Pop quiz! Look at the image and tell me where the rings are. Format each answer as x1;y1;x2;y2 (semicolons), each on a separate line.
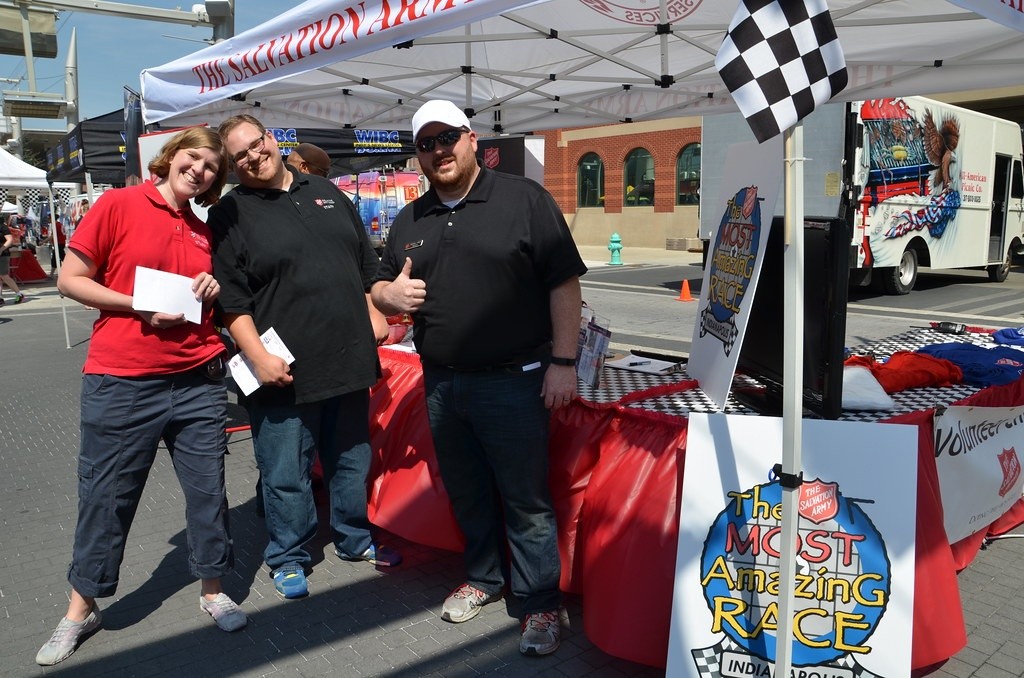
209;286;213;290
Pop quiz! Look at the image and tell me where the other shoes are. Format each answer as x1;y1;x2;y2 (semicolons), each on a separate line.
46;276;54;281
256;501;265;516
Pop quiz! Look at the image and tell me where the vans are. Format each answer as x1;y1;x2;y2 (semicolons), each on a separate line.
849;95;1024;296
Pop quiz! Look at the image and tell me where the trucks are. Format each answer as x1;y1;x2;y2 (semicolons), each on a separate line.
33;195;103;244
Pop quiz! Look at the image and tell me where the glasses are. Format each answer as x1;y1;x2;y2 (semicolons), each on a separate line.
304;160;329;179
230;132;265;167
415;129;469;153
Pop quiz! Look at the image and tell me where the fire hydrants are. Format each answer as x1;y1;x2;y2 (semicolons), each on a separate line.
608;233;623;264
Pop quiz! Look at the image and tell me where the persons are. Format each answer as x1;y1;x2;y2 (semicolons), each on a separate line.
36;126;246;666
0;222;24;306
39;214;66;280
371;90;586;656
287;143;330;178
206;113;402;598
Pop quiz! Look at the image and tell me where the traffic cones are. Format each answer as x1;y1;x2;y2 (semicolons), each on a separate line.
675;280;696;301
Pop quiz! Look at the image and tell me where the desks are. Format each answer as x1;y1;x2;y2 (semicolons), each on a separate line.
312;326;1024;670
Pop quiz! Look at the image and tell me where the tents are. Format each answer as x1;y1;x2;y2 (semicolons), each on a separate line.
139;1;1024;678
47;108;125;270
0;146;103;271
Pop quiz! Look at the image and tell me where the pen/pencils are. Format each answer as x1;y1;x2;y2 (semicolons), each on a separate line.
626;361;651;366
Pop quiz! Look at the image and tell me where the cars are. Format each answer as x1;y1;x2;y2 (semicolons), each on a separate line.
8;216;35;245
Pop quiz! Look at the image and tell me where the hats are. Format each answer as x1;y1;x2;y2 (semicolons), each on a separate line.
295;143;331;178
412;100;473;147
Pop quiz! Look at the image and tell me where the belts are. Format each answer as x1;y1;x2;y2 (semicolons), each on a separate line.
425;362;522;376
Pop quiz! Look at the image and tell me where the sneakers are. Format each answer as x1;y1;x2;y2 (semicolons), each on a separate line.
0;299;5;307
519;608;560;656
15;294;24;303
335;542;401;567
273;567;307;598
36;599;102;666
440;583;503;623
200;590;247;633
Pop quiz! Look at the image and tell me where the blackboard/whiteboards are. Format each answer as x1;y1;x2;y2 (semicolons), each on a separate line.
699;102;846;239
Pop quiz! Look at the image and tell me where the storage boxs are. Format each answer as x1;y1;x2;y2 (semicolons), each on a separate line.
1;246;21;258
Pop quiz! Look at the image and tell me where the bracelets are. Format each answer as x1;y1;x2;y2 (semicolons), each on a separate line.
551;356;576;365
